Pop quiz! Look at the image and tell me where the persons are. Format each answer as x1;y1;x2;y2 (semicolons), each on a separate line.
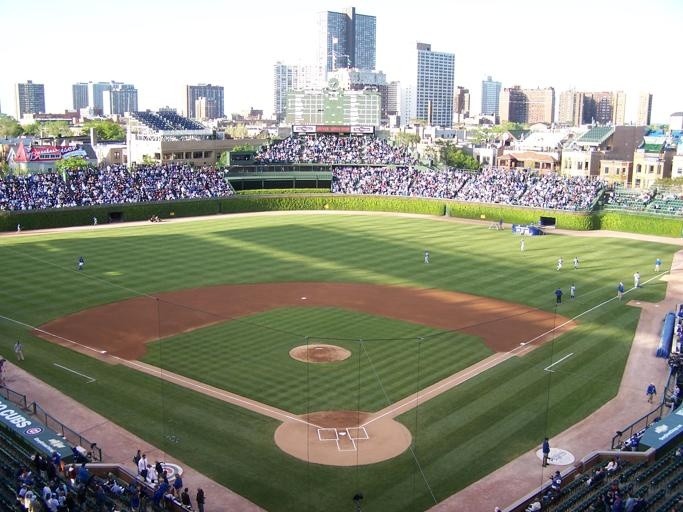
633;272;640;288
0;338;207;511
573;256;579;268
557;257;564;270
0;160;233;212
17;224;21;231
494;307;683;512
654;258;661;271
425;250;428;263
570;284;576;298
93;217;98;226
617;282;624;301
255;134;419;166
520;239;525;251
333;166;683;212
554;287;563;305
78;256;84;270
